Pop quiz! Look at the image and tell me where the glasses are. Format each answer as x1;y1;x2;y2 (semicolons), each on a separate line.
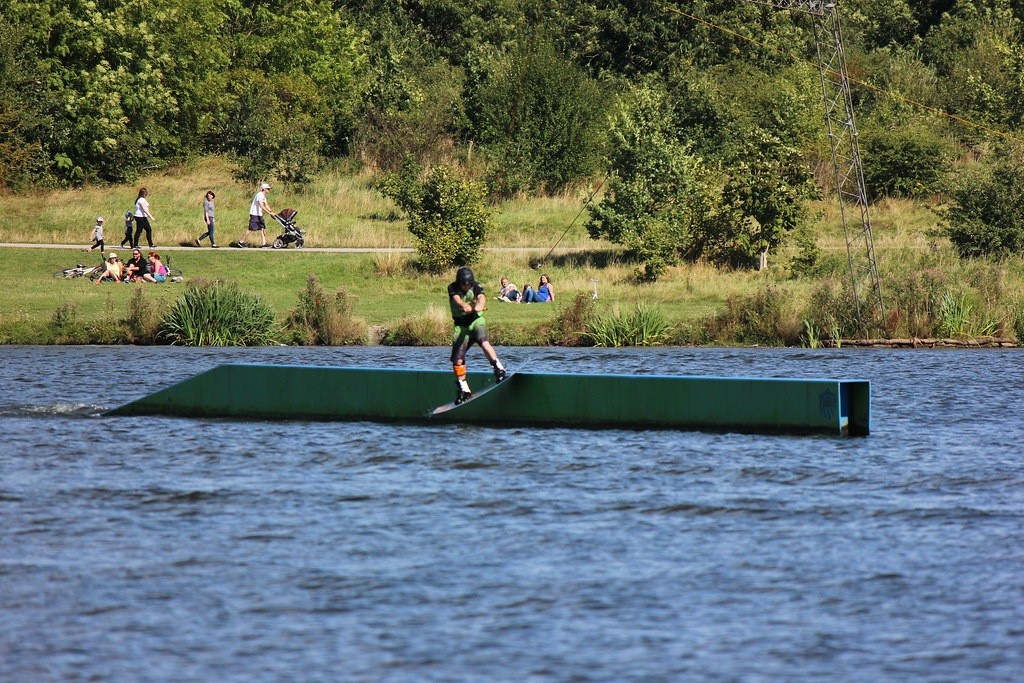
148;256;150;259
133;253;140;255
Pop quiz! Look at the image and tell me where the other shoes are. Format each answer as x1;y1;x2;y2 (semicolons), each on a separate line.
490;358;506;384
212;245;219;248
455;379;471;405
196;239;200;247
86;243;167;284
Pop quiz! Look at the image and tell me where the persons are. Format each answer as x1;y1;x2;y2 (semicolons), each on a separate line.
237;184;276;248
446;267;507;406
86;217;105;253
195;190;219;248
497;277;523;304
521;273;555;304
123;249;147;283
290;221;306;234
522;284;532;295
120;211;137;249
143;252;167;282
133;187;158;249
95;252;124;284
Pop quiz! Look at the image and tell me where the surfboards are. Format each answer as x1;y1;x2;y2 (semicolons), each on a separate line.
432;356;532;415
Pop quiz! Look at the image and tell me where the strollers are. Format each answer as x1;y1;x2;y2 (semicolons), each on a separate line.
271;208;307;248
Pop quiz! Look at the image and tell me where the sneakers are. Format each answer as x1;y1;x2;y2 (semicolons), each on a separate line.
498;296;531;304
238;241;247;248
262;244;272;248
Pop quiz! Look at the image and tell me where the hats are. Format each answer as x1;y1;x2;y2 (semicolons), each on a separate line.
125;212;131;217
97;217;103;221
261;184;271;189
109;253;117;258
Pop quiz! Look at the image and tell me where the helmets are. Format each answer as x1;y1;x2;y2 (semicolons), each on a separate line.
456;267;474;285
